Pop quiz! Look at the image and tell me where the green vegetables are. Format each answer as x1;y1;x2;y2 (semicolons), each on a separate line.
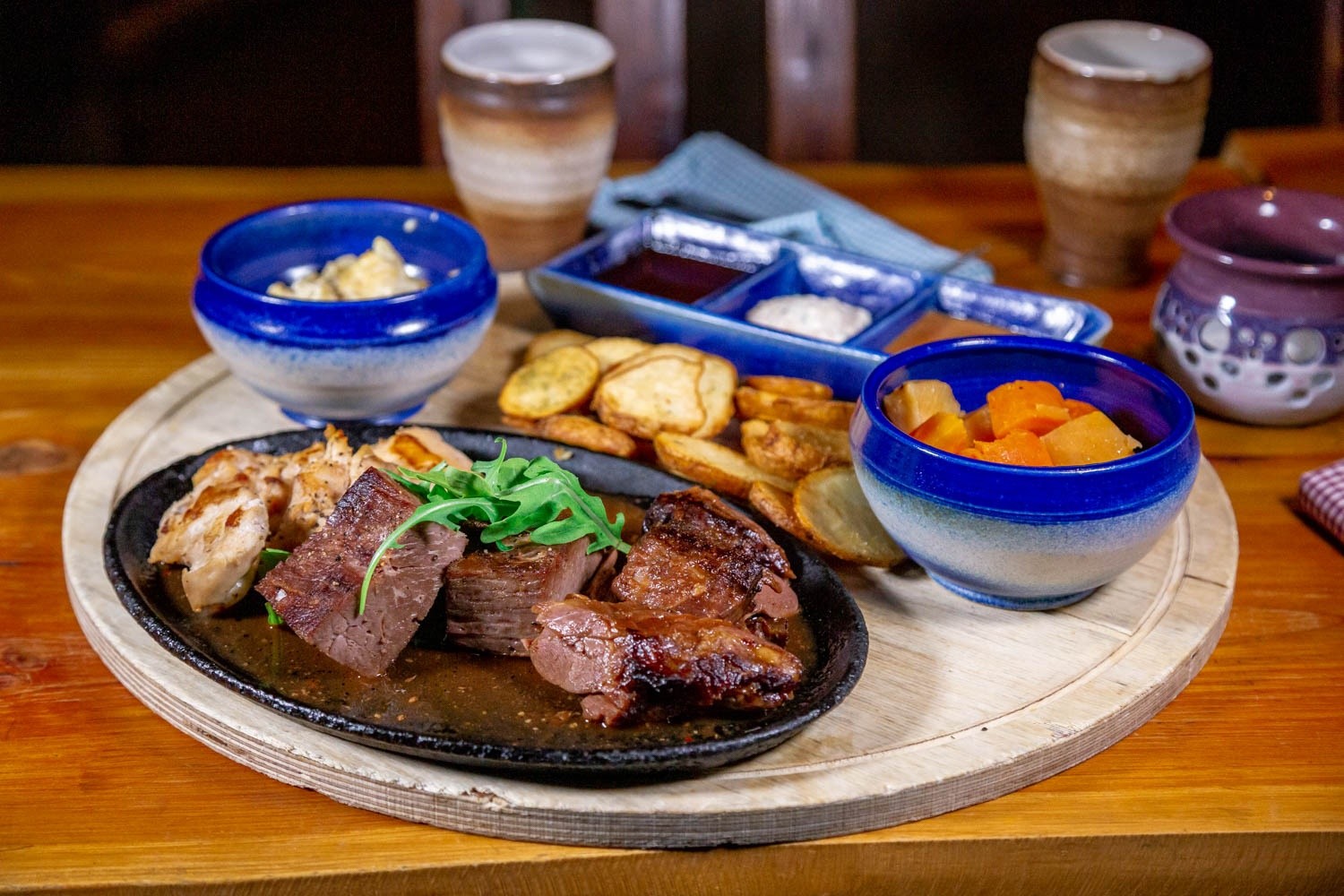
251;432;631;623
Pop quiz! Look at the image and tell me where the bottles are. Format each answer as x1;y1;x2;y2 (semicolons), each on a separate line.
1152;186;1344;430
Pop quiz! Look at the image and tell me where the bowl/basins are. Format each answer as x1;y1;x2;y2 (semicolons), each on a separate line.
525;201;1114;404
188;197;497;427
848;333;1200;609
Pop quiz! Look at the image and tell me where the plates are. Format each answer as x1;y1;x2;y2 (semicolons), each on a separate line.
103;427;870;785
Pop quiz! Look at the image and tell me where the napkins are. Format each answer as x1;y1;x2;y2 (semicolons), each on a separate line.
587;133;993;285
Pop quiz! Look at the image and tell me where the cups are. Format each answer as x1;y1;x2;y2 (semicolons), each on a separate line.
1024;20;1215;288
437;17;621;273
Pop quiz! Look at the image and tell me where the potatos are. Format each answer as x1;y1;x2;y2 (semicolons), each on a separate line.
494;331;910;568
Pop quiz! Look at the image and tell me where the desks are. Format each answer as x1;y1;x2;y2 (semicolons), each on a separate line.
0;161;1344;896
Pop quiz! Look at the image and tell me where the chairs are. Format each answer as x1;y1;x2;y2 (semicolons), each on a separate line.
416;0;860;163
